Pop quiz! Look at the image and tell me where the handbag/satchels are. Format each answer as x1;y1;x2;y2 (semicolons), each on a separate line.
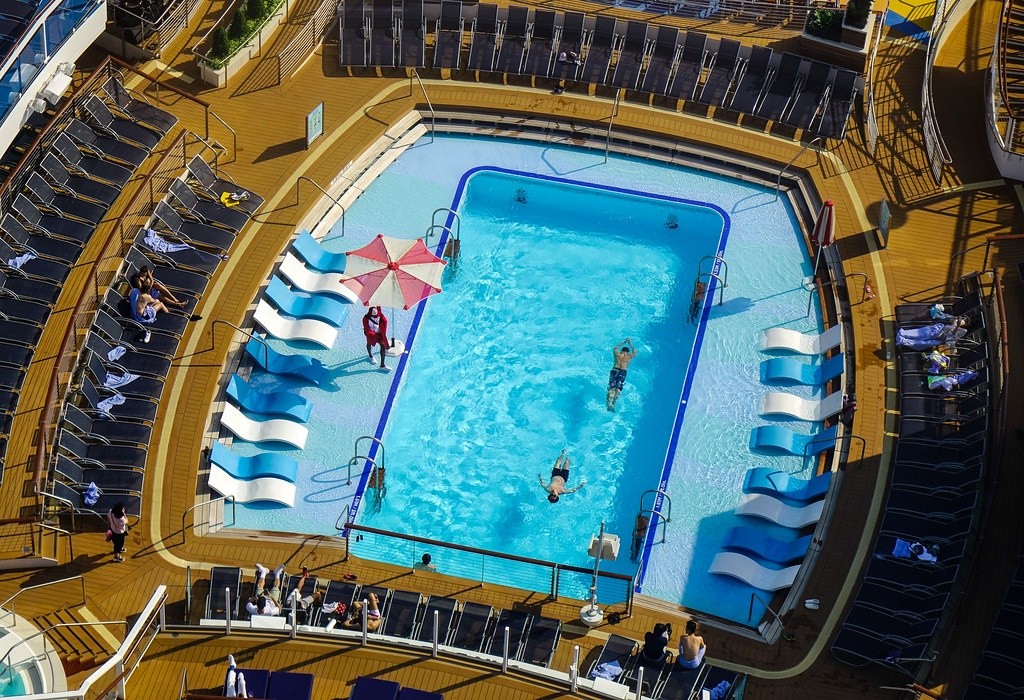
124;526;129;536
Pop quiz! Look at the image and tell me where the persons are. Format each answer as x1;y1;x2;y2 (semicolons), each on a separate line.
226;654;248;698
642;623;672;664
606;338;638;413
363;307;392;371
895;315;970;350
129;266;188;323
230;190;248;201
631;515;649;560
343;592;380;631
538;449;585;503
677;621;706;669
108;502;129;562
553;85;565;93
559;50;581;65
416;553;436;572
247;563;322;616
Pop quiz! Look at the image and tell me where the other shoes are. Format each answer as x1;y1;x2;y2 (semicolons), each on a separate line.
805;599;819;604
369;356;378;364
255;564;270;574
343;573;359;581
303;567;308;578
805;604;819;609
274;564;284;575
380;365;392;371
113;555;124;562
783;632;795;641
665;623;672;635
120;547;126;552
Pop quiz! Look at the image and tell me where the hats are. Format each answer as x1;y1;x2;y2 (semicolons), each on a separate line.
369;306;377;315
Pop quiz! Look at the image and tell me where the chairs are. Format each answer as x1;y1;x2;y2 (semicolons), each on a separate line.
1;1;1024;700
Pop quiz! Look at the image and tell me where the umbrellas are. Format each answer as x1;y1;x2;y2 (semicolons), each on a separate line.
812;200;835;282
339;236;448;348
